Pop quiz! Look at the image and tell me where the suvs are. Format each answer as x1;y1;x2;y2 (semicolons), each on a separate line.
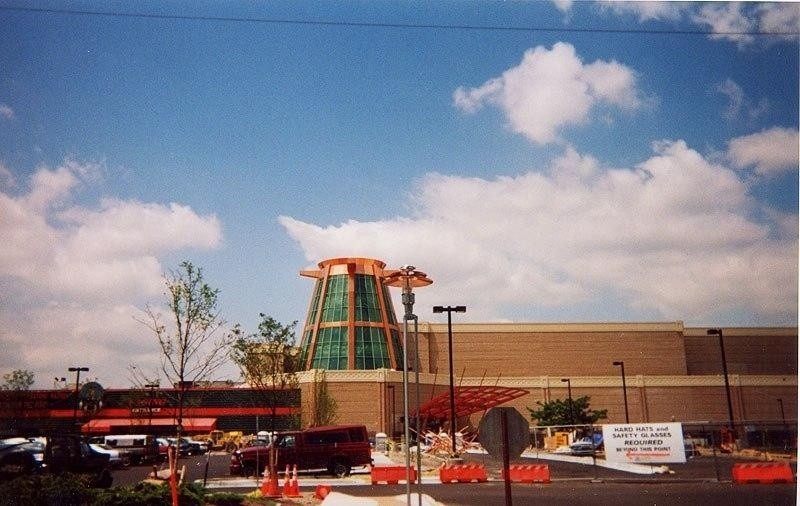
227;422;372;478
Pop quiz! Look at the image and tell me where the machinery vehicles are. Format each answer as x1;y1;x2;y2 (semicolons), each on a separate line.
194;429;254;452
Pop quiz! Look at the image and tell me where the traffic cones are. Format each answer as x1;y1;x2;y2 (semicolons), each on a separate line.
291;465;303;498
260;467;272;498
282;462;292;498
269;465;281;498
315;485;332;499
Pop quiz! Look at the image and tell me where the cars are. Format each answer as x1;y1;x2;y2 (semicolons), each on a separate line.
571;436;597;449
0;431;212;483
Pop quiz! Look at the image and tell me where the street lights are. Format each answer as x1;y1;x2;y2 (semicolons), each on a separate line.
55;377;67;382
777;398;789;447
558;379;575;434
143;384;159;433
430;304;467;458
706;328;739;446
67;366;90;444
613;360;632;423
382;262;435;505
